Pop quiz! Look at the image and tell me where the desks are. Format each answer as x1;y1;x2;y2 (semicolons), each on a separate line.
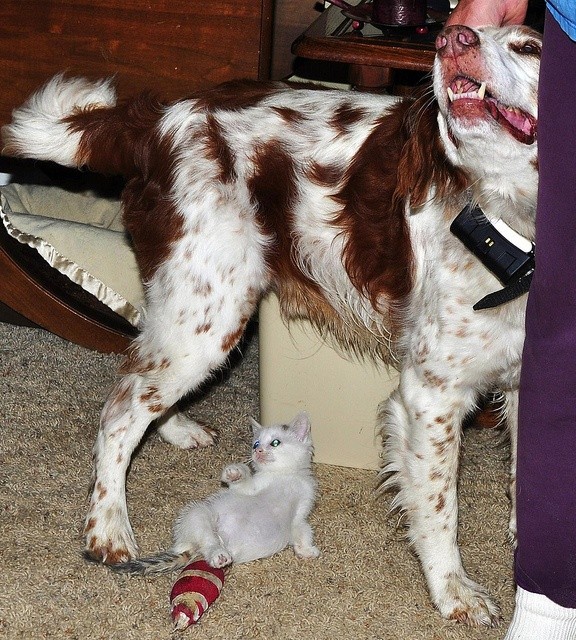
290;0;466;91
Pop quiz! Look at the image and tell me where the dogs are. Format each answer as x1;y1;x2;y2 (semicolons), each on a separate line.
2;21;545;630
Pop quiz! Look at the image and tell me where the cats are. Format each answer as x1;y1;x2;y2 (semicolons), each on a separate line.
107;411;323;579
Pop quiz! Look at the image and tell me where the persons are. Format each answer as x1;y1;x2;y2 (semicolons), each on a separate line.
444;1;576;640
